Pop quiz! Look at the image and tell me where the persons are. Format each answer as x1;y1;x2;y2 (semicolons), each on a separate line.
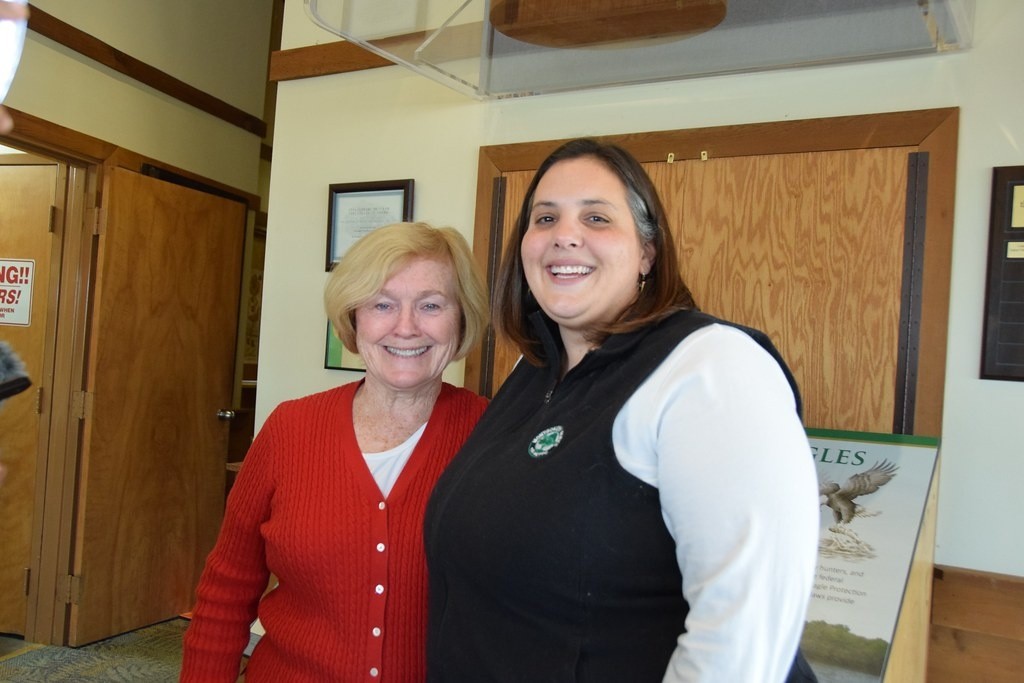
176;222;490;683
420;139;817;681
